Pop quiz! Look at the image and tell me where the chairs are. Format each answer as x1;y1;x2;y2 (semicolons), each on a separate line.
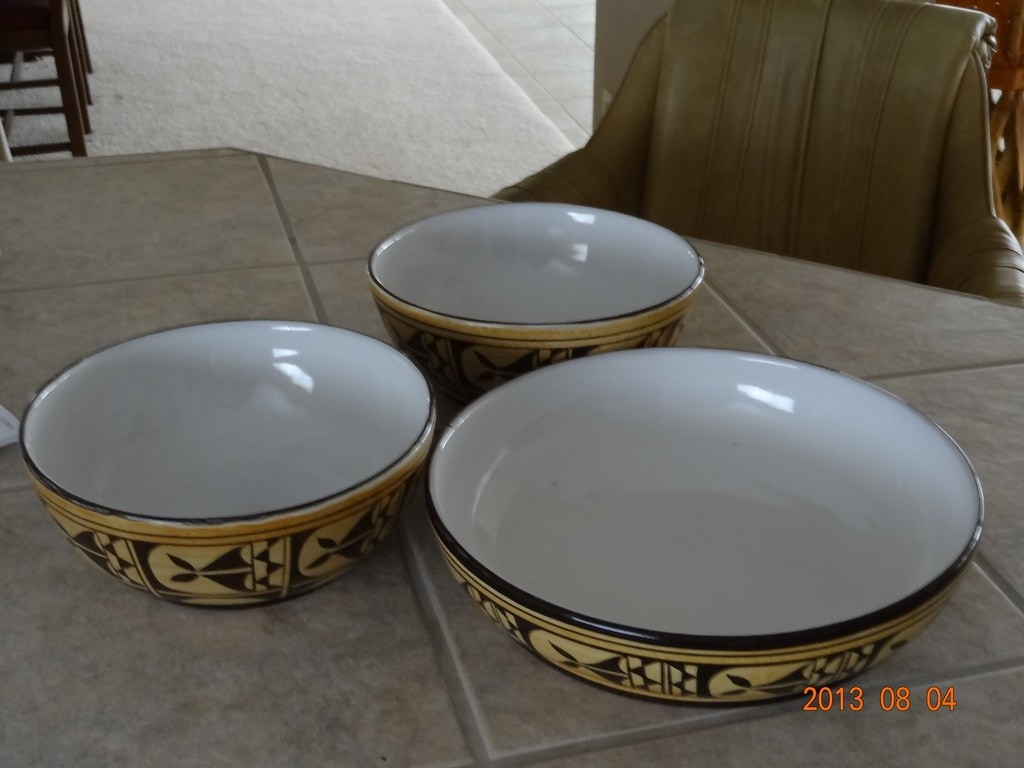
489;0;1024;304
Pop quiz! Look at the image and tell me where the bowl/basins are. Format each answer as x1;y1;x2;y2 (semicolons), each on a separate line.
19;319;435;612
367;201;706;400
423;344;989;711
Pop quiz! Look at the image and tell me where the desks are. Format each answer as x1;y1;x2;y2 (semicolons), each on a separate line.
0;148;1024;768
